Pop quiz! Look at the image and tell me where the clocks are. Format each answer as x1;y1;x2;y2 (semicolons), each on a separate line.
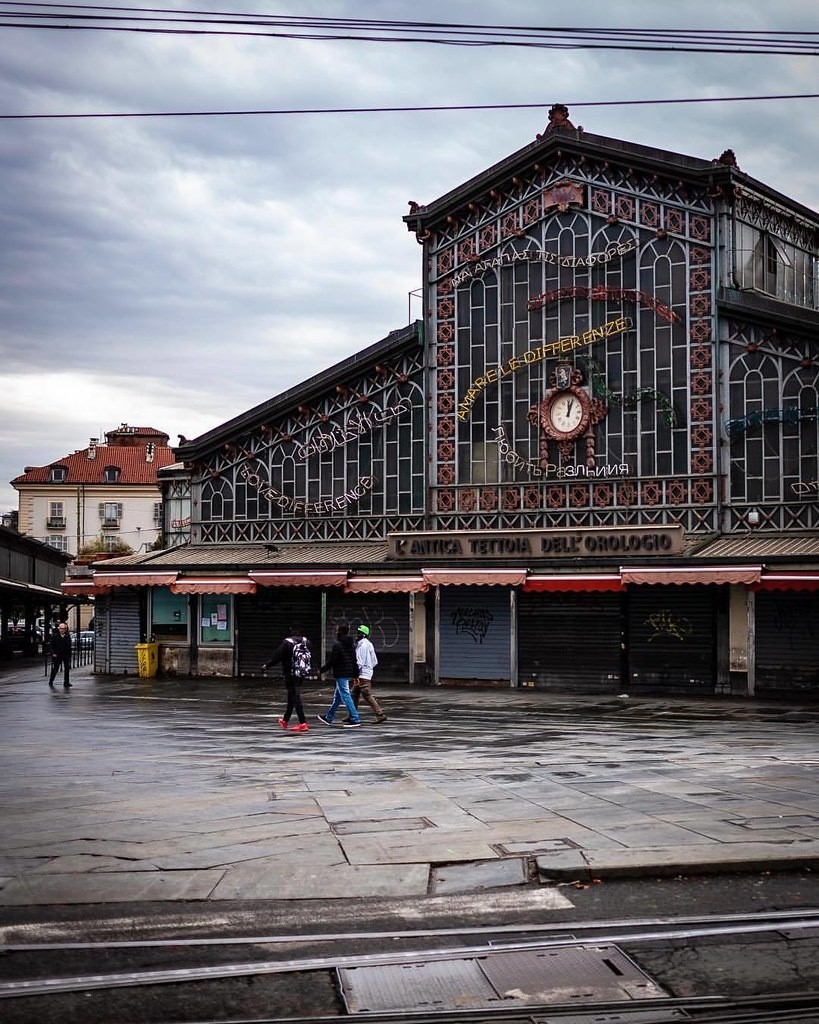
550;392;585;433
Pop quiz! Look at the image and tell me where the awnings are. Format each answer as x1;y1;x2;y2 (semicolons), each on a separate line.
93;570;178;586
522;572;626;592
60;580;112;596
746;571;819;593
248;569;350;586
622;565;763;584
344;575;429;594
170;577;257;594
421;568;528;586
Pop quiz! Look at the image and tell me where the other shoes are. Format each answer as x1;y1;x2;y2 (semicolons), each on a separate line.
371;714;387;724
48;682;54;688
342;717;352;722
64;683;73;688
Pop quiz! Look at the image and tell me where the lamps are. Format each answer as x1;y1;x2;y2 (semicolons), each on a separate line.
263;543;280;558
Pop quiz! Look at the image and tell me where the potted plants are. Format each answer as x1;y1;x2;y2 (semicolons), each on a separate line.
77;531;135;561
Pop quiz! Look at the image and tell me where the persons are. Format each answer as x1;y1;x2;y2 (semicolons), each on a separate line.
317;624;361;728
12;625;21;632
48;623;73;688
261;623;314;731
342;624;387;724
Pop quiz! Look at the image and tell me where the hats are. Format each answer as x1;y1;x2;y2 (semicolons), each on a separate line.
357;625;370;636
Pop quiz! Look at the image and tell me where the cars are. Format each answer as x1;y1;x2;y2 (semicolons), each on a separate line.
6;616;95;655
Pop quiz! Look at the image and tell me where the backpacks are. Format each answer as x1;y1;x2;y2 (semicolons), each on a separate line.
284;636;312;677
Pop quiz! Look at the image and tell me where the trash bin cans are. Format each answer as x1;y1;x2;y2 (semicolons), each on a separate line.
134;643;160;678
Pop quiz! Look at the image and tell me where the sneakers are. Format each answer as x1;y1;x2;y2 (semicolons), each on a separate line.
317;715;341;721
343;721;361;727
278;719;289;728
290;722;309;731
